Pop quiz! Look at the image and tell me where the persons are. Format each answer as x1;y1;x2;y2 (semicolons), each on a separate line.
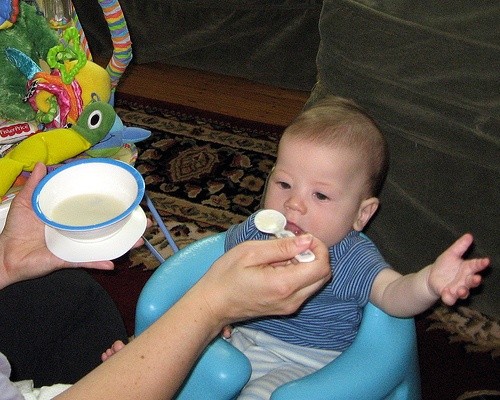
0;162;332;400
102;95;490;400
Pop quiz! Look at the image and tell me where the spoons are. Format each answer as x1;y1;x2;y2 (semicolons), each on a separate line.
254;208;316;263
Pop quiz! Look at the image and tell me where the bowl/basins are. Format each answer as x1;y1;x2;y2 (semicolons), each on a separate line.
31;158;145;242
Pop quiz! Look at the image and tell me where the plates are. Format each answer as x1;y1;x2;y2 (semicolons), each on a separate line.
44;205;147;262
